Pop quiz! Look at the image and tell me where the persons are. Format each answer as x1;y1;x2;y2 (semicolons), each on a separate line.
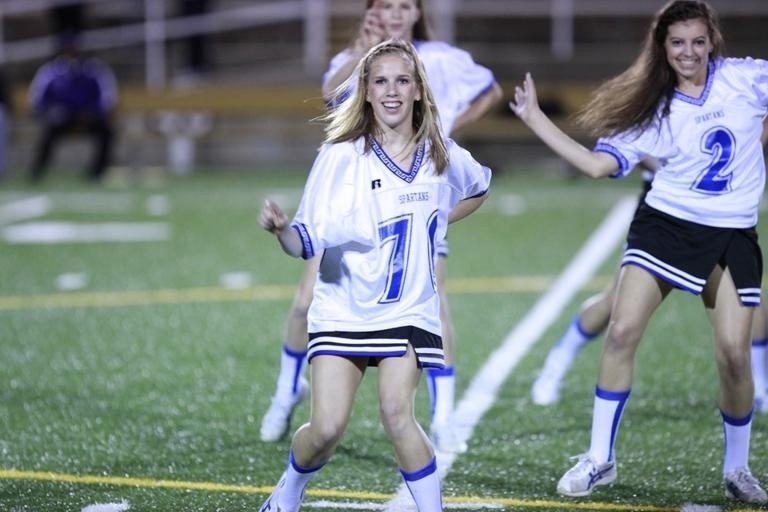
258;34;490;509
256;2;503;457
161;54;223;173
525;252;766;416
509;0;766;508
25;34;116;183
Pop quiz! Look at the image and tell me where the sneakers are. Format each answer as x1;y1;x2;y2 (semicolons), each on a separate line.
258;472;308;512
557;448;618;498
259;378;309;441
427;423;466;454
720;468;767;506
533;347;573;406
